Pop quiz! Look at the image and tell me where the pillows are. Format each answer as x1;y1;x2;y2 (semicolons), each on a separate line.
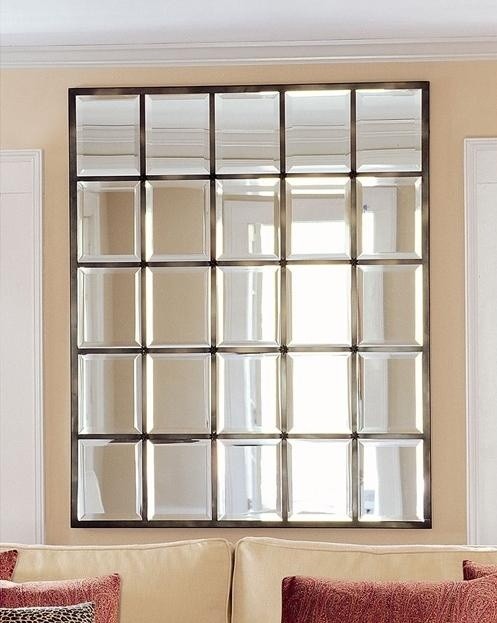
0;547;123;623
280;558;497;623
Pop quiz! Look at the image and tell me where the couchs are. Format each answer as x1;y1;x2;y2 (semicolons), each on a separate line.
0;534;497;623
